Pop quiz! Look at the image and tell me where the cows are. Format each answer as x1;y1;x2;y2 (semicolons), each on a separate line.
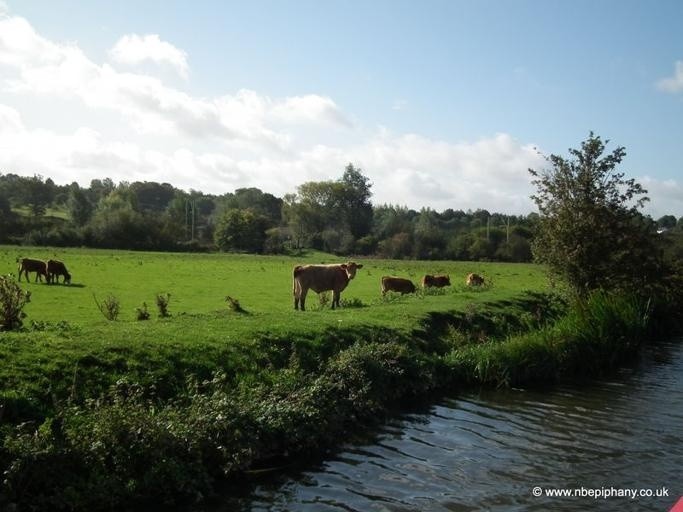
466;273;484;286
18;258;52;283
293;262;364;311
421;274;452;289
47;259;71;285
381;276;417;297
